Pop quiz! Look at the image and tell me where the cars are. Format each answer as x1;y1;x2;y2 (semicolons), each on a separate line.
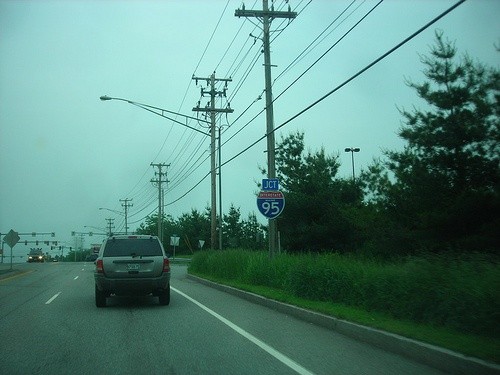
52;258;58;262
27;249;45;263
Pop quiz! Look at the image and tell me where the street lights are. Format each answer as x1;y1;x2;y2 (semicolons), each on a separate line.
345;146;360;180
85;225;112;237
100;94;222;250
99;207;128;235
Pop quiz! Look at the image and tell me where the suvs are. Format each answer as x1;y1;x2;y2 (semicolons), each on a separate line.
93;233;172;308
